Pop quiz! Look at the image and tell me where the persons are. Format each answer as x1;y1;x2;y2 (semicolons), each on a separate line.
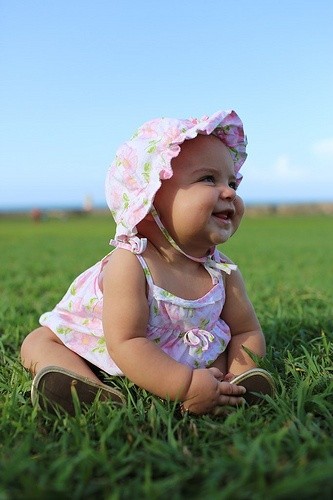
20;108;277;418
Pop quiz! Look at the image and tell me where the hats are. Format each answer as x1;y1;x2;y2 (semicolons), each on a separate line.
104;108;249;245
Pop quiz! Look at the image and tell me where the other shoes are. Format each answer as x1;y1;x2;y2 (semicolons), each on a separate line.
226;369;277;411
30;367;128;417
